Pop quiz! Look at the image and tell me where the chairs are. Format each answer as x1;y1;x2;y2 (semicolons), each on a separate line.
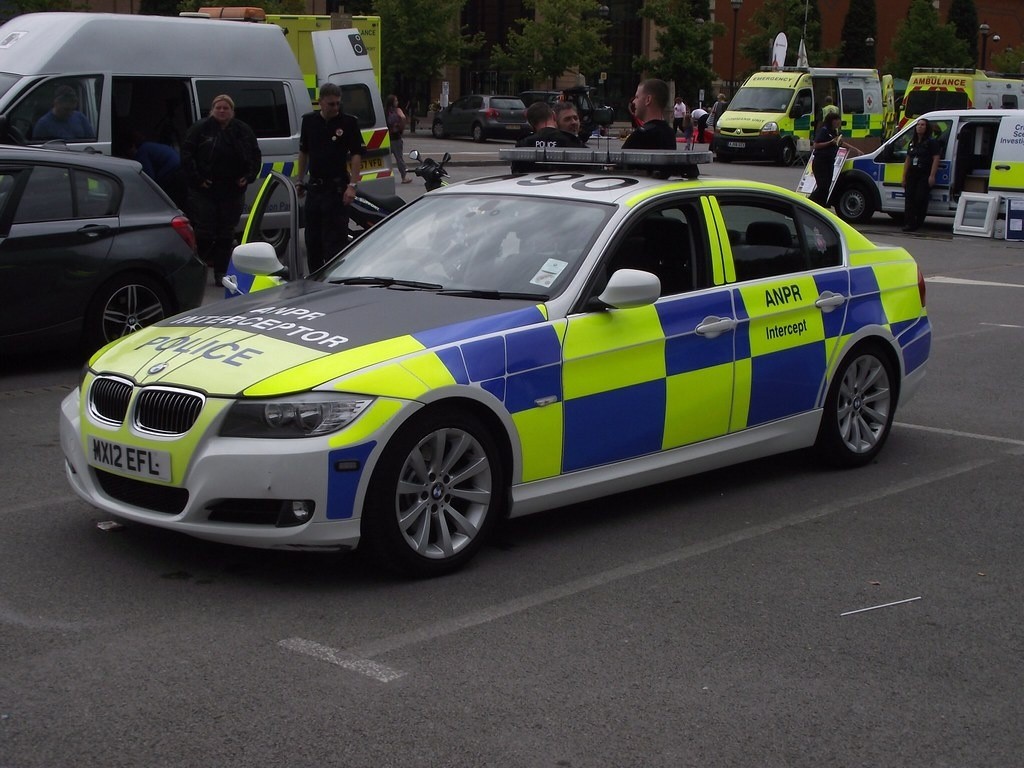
32;170;92;219
791;136;810;168
746;221;793;249
639;216;695;297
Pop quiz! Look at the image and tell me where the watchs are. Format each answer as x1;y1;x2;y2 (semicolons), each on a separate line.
347;183;359;192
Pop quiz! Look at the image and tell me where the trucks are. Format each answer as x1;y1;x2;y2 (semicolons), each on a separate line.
180;7;382;110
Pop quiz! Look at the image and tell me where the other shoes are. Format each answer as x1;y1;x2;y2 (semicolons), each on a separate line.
401;178;412;183
214;273;226;287
416;119;420;124
902;224;917;232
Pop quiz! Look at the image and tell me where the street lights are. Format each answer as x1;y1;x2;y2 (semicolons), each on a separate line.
730;0;745;102
552;2;610;91
979;22;1014;74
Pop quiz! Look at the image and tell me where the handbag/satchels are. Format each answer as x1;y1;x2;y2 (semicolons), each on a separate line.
705;115;714;126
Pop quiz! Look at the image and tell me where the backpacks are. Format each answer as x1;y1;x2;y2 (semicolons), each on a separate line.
387;107;401;134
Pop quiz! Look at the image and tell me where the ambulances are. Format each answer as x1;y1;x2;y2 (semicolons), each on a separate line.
898;65;1024;135
709;66;896;166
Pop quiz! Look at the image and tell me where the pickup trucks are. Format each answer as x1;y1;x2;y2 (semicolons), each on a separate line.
520;89;614;144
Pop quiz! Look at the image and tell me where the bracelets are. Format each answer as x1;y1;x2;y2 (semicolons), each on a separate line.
295;177;303;187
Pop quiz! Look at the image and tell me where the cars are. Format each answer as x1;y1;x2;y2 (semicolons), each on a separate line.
0;139;204;377
432;94;533;142
55;157;933;576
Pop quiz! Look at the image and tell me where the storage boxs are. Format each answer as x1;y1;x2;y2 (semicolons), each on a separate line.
797;139;810;151
964;174;989;193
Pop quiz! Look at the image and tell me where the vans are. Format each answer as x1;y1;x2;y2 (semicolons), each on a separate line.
832;109;1024;225
0;12;318;259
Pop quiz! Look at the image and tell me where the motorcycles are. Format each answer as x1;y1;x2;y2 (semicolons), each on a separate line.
345;148;453;244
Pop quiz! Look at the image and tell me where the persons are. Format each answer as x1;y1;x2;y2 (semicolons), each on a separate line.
32;85;95;196
182;94;262;287
511;101;582;174
120;130;182;200
808;96;841;208
294;83;366;274
385;94;412;184
621;79;677;150
405;96;420;125
902;118;941;232
673;93;728;151
628;95;638;132
